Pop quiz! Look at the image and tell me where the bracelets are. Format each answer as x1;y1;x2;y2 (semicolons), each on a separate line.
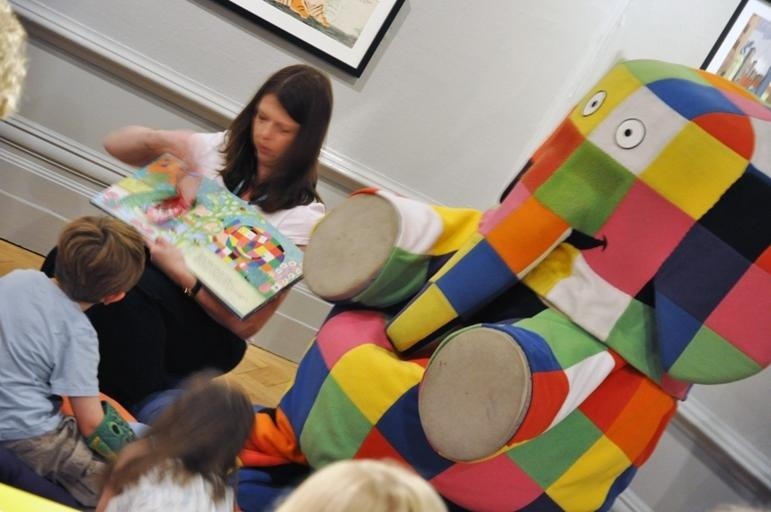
144;130;158;152
182;278;201;298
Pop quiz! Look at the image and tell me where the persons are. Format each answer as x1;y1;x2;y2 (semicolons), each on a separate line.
36;64;337;424
272;455;451;511
103;376;254;512
0;210;145;510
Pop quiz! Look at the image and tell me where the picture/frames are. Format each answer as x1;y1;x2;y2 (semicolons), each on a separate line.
698;0;771;110
210;0;405;79
